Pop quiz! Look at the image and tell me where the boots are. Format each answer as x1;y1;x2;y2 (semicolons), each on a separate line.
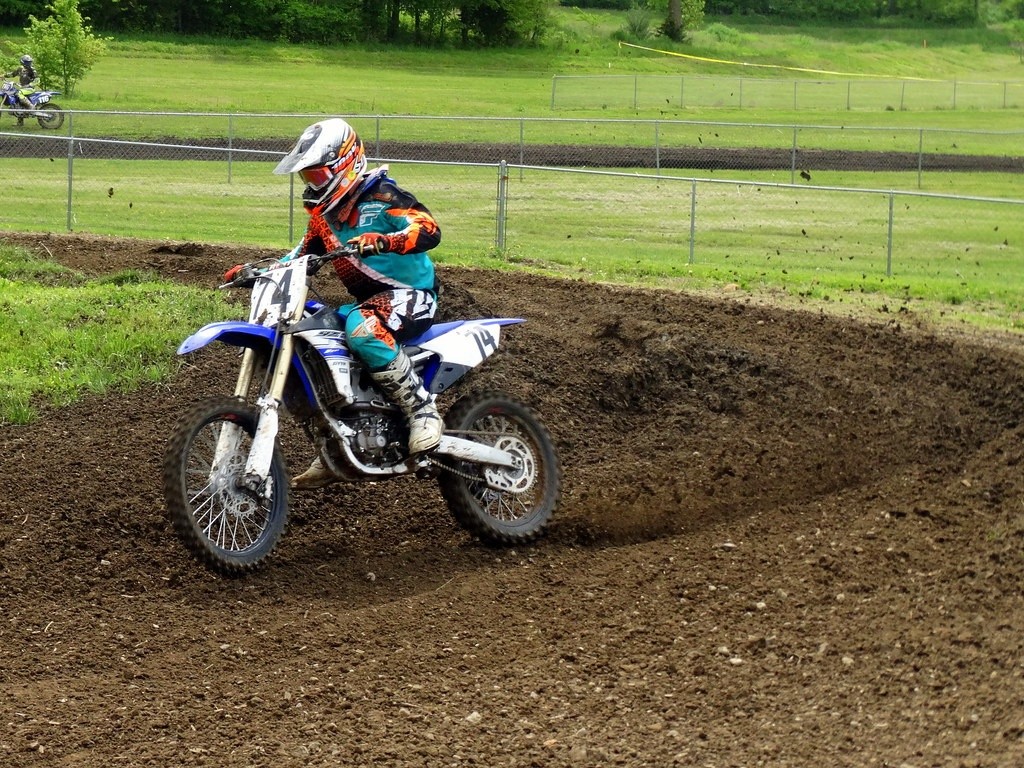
293;452;333;485
370;347;445;454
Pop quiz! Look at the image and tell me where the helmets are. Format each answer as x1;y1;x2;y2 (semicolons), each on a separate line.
272;118;367;218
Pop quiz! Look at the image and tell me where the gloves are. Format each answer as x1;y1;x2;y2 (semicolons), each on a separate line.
346;232;389;256
225;264;243;281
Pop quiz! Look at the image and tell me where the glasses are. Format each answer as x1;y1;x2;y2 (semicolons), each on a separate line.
297;135;360;191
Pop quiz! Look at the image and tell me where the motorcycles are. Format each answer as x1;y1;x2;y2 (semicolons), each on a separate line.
0;73;65;129
161;240;561;577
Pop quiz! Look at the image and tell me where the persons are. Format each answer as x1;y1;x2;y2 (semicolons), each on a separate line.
0;55;42;126
222;118;447;490
